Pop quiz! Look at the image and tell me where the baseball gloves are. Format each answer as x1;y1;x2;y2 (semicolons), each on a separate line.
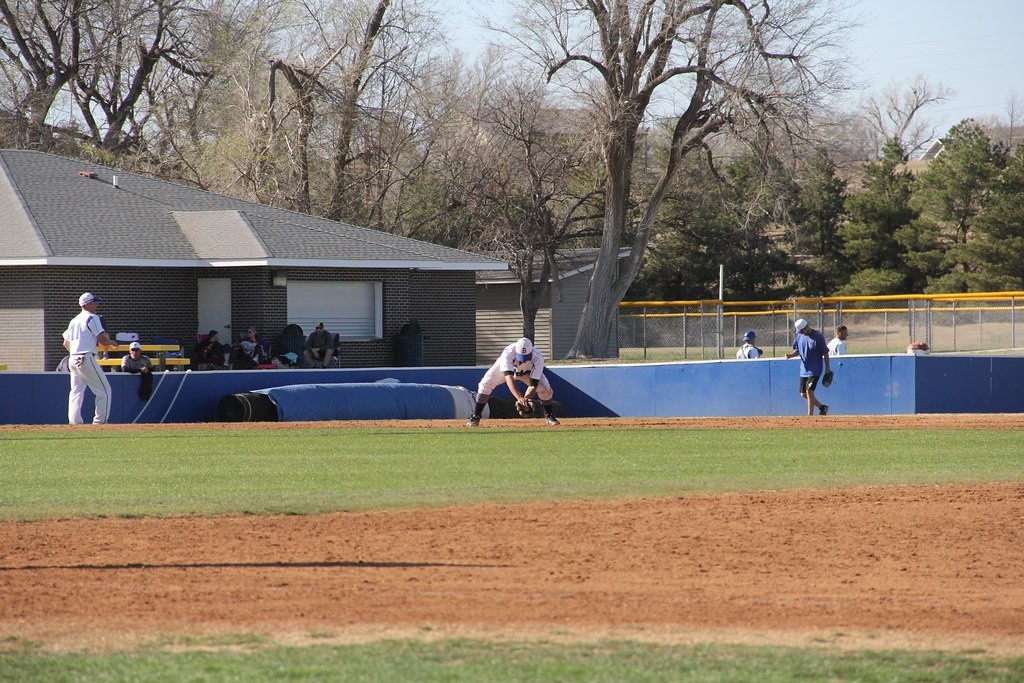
514;398;536;419
822;371;833;387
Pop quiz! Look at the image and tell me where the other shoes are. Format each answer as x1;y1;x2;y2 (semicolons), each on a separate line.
818;405;829;415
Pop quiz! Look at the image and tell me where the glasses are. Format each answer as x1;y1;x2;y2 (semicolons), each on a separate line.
246;330;253;334
316;326;323;329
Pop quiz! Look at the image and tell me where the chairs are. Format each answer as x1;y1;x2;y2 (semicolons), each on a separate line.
115;331;140;357
238;334;270;368
320;332;341;366
152;337;185;373
196;333;222;370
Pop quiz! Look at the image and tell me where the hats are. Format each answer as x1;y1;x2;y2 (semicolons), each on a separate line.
129;342;141;350
515;338;532;361
78;292;101;307
742;332;755;341
795;318;807;333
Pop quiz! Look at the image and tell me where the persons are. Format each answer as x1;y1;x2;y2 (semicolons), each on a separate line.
785;319;833;415
62;292;118;424
737;331;758;359
468;337;560;427
827;326;848;356
121;342;153;373
304;323;335;368
195;328;261;370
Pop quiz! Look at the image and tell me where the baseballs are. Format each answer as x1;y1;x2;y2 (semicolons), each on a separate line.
520;405;529;412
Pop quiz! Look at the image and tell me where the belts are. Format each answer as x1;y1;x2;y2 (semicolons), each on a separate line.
79;353;95;356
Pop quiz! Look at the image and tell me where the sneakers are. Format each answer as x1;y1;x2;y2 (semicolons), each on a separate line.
467;415;481;427
545;417;559;426
